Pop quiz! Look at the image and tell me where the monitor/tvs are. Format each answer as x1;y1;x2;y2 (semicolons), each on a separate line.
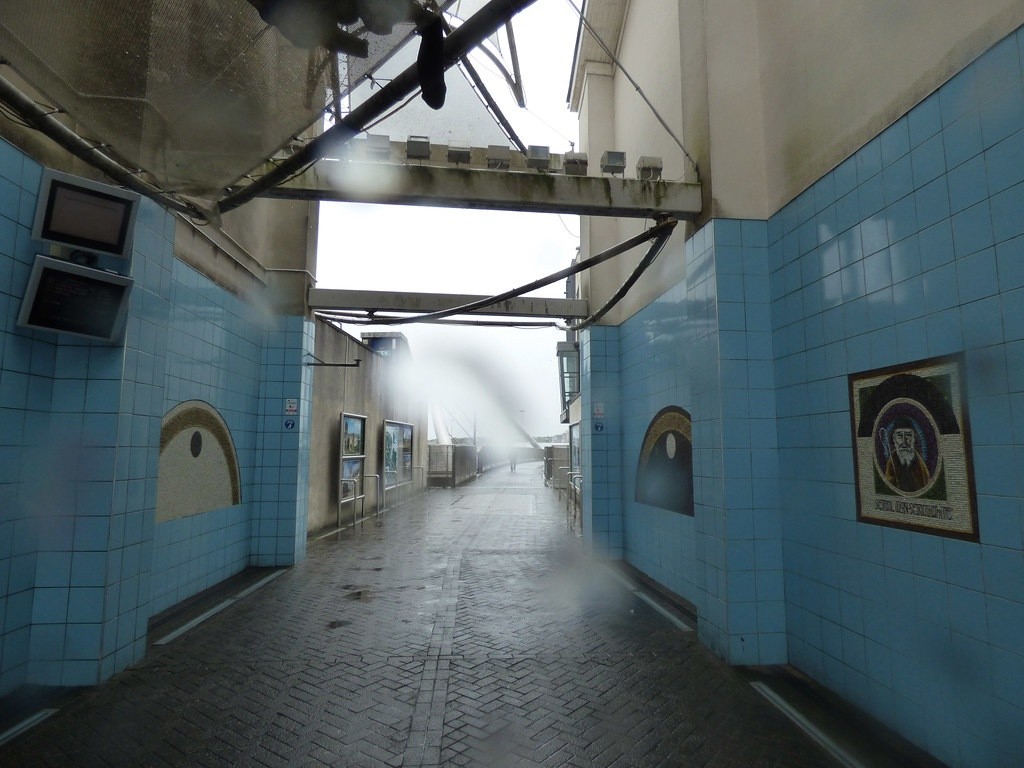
30;166;142;261
15;253;135;344
